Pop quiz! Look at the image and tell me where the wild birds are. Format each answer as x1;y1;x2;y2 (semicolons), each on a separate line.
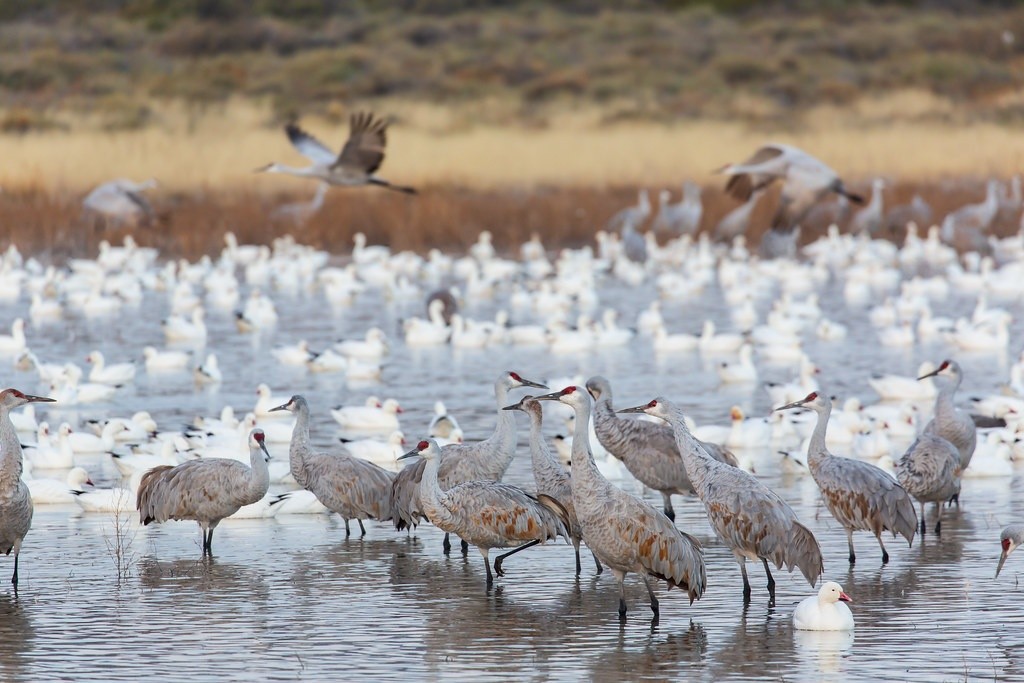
251;110;416;194
0;147;1024;634
